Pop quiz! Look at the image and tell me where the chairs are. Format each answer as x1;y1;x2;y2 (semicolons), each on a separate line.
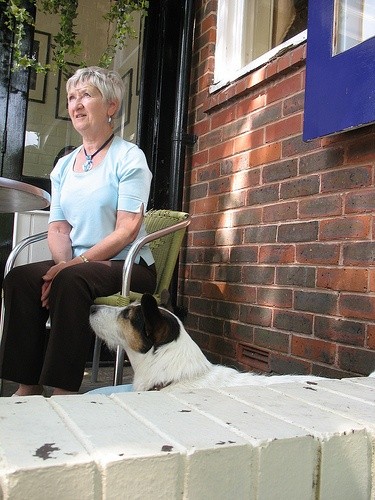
5;208;192;387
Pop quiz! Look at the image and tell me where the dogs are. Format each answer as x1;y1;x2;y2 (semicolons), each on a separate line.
90;294;329;392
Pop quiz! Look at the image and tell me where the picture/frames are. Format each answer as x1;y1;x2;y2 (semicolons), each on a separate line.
26;30;52;104
113;68;134;133
54;62;88;121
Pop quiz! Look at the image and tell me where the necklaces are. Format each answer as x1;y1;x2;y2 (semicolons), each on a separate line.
82;135;114;171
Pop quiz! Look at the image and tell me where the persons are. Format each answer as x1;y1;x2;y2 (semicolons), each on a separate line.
0;66;153;397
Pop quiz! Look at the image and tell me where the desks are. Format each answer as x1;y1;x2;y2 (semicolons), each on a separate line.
0;177;50;214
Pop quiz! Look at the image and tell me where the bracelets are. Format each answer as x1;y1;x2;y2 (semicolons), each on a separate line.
78;252;90;264
